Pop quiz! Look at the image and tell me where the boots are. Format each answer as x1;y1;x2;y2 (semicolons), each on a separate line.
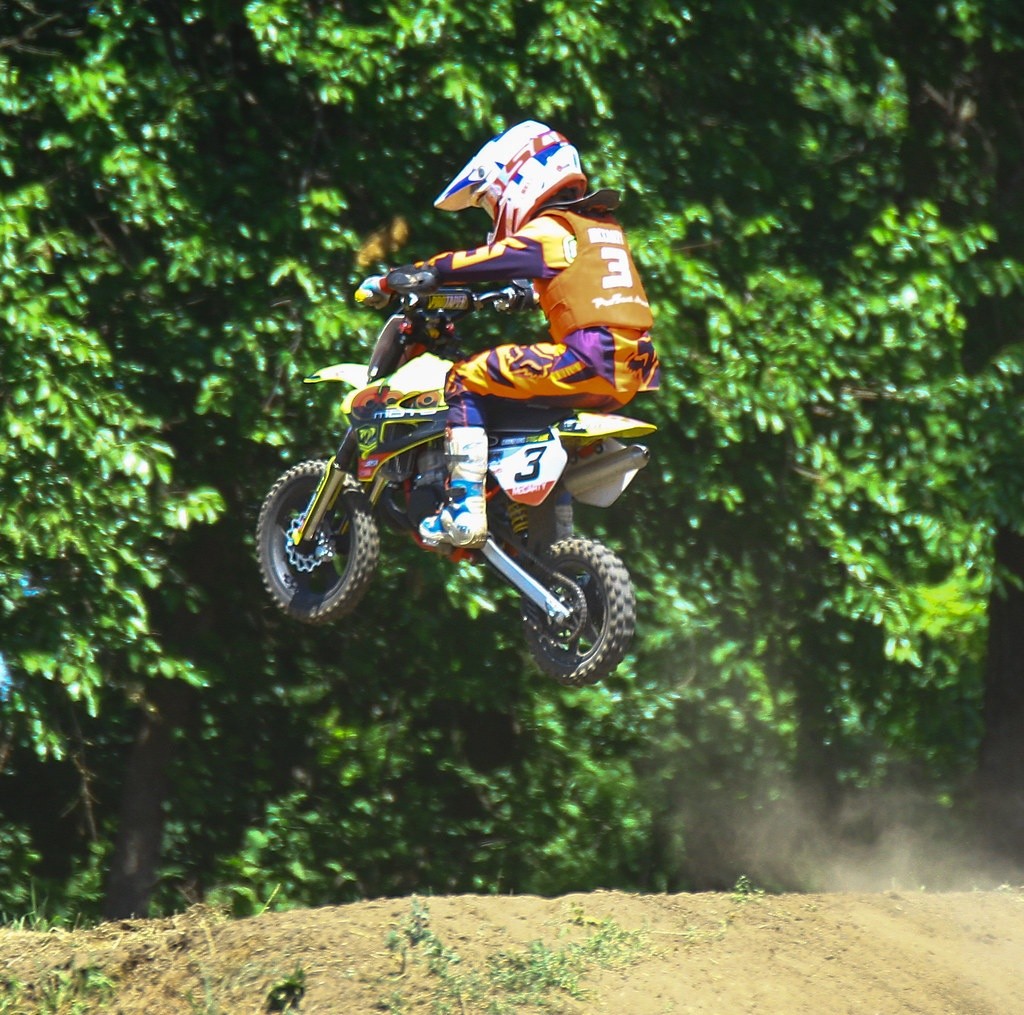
419;427;489;547
557;490;574;539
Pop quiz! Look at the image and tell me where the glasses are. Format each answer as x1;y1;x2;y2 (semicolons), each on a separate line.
477;130;569;222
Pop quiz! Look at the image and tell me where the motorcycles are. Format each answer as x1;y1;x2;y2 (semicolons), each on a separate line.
254;276;659;689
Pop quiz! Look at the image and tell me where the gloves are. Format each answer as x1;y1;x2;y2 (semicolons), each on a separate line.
494;283;540;314
359;276;395;310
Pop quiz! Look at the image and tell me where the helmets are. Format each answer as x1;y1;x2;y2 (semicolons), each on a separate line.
434;120;588;246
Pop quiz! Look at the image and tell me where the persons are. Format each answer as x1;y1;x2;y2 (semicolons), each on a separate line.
352;116;663;549
